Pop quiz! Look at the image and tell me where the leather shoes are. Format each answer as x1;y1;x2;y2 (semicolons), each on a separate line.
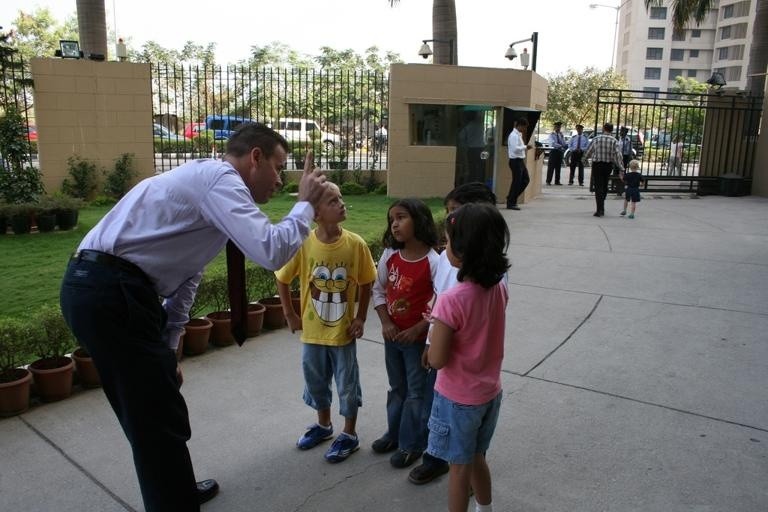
196;480;217;505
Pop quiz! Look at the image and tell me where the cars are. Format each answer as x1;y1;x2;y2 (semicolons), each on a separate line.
180;121;206;139
21;123;38;142
534;133;555;157
152;123;191;142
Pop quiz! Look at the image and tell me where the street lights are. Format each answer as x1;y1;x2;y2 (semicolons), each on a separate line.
588;1;622;123
415;39;455;65
501;30;539;71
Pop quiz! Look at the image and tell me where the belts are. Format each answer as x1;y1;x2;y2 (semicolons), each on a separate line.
72;249;147;280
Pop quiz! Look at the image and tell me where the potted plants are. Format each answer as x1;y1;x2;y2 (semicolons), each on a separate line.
0;193;82;235
326;143;350;169
290;148;314;170
23;305;80;401
0;314;34;418
176;265;301;361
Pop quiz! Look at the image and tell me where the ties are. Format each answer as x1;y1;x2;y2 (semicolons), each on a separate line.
577;135;580;148
225;239;247;347
557;133;559;144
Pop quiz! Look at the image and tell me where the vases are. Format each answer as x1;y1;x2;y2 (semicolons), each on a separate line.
72;342;99;387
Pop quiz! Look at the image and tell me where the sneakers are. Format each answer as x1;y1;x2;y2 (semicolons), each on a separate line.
371;437;448;485
323;433;359;463
296;423;334;450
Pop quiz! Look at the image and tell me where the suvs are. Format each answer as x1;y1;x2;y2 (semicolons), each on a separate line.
266;117;343;158
560;126;645;169
204;114;257;141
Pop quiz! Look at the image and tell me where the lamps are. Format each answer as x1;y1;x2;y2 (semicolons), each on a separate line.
705;72;727;90
58;39;81;59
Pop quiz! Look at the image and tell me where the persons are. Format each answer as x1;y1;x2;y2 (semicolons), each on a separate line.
373;199;440;468
408;183;496;498
457;110;486;183
425;202;511;511
566;125;589;187
546;121;568;186
378;124;388;152
59;121;329;512
274;181;377;464
668;135;683;176
507;118;533;210
580;123;644;219
